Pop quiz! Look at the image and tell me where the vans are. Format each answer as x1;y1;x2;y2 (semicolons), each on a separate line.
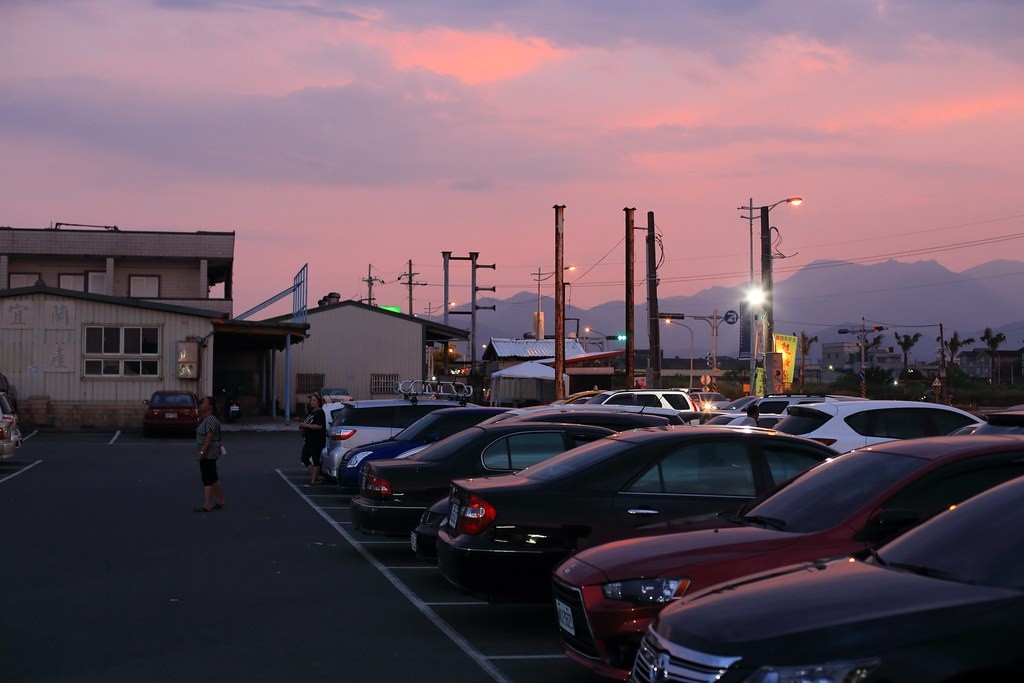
552;385;700;417
323;400;480;479
0;392;22;459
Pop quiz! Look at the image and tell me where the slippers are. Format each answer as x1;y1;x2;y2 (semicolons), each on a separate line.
193;503;224;512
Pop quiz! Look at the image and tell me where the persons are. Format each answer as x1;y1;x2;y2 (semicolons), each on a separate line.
191;395;224;512
297;393;326;488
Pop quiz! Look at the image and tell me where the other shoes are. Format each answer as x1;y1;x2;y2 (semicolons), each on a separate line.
304;479;321;487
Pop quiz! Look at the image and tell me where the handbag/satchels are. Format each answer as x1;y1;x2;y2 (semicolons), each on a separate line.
220;445;227;455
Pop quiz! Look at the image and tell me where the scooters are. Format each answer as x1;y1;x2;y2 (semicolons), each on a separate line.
222;386;242;423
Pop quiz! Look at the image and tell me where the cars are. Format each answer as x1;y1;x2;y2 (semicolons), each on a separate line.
141;390;202;435
553;435;1024;679
352;421;616;522
709;397;759;415
689;391;730;411
436;427;842;597
630;479;1024;683
318;387;353;403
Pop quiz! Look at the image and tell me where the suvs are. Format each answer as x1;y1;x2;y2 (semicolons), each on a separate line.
757;393;871;418
774;400;988;453
339;407;516;491
397;407;688;479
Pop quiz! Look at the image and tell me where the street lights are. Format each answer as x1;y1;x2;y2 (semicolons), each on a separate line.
746;197;801;390
531;265;572;339
